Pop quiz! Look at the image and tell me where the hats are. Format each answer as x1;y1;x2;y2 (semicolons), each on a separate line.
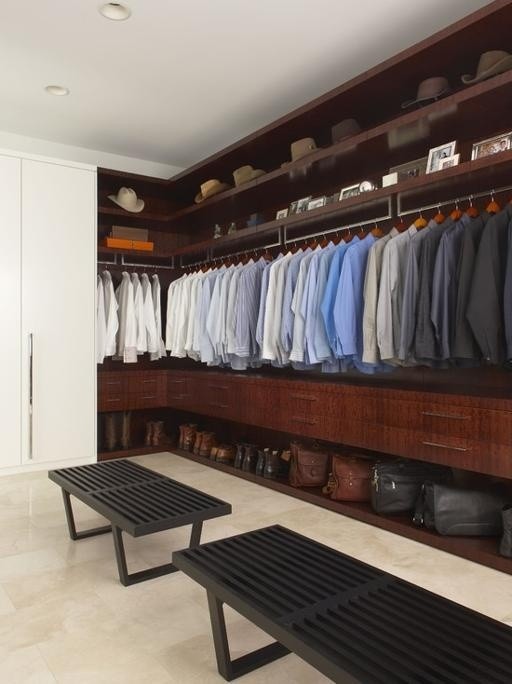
106;181;148;214
458;46;512;85
397;73;456;110
229;163;273;189
322;113;367;147
191;177;232;204
278;134;328;170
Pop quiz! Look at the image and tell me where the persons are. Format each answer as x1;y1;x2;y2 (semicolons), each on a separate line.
439;137;507;164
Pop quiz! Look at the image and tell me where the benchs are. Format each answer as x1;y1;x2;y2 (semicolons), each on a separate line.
170;520;511;684
45;457;233;588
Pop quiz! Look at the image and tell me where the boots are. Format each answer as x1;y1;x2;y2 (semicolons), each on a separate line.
142;418;155;448
241;443;260;472
118;414;142;450
198;430;217;456
192;428;208;456
177;421;189;449
102;412;121;451
182;423;199;451
233;441;250;469
254;446;272;476
151;419;177;448
263;448;290;479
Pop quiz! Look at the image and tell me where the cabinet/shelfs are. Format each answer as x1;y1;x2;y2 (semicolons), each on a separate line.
1;147;101;476
97;1;512;573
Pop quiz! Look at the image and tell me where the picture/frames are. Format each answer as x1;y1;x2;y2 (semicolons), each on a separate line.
469;130;512;160
388;155;426;185
423;139;457;175
438;152;460;169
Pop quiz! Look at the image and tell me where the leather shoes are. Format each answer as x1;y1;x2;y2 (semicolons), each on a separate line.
209;441;236;464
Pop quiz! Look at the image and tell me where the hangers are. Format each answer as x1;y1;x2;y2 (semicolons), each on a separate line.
182;189;501;276
95;256;159;282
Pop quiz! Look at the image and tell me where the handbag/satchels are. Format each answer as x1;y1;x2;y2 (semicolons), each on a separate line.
367;455;427;516
410;471;504;539
497;502;512;559
328;447;379;504
287;437;333;489
